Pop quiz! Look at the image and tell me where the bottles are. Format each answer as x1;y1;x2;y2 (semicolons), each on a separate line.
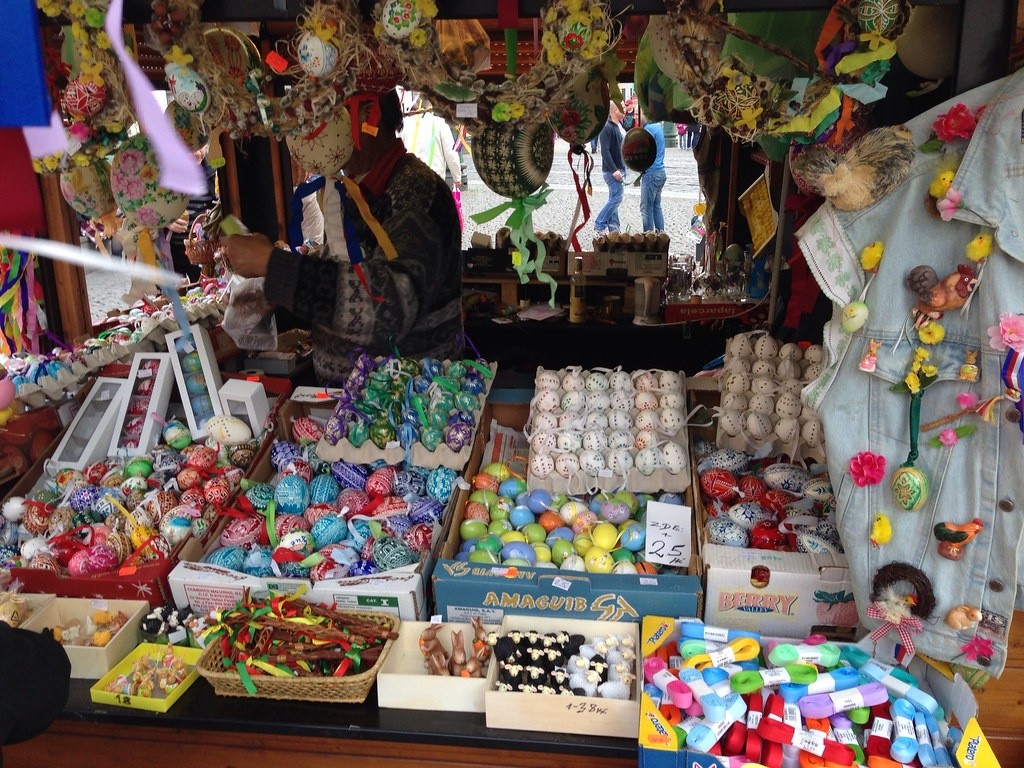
570;257;587;322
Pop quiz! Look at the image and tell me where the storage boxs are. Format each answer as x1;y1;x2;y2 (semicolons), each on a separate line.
167;387;484;621
8;387;288;607
483;613;640;737
429;401;704;620
471;232;490;248
638;614;1003;768
23;599;149;679
567;250;668;280
374;618;501;714
682;374;870;641
1;588;56;630
542;255;562;274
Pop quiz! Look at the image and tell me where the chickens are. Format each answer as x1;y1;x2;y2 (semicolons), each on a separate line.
906;264;978;313
934;517;984;555
869;582;918;625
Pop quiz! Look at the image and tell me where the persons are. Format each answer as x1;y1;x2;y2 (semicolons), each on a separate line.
220;68;464;391
162;99;700;283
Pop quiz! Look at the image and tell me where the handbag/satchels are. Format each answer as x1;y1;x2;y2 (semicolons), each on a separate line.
452;184;464;233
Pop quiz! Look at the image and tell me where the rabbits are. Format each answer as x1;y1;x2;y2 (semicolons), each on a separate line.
419;616;491;678
944;605;983;631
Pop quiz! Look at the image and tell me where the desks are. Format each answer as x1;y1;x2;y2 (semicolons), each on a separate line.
462;269;634;310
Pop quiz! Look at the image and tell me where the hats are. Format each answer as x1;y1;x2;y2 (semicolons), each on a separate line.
626;100;634;104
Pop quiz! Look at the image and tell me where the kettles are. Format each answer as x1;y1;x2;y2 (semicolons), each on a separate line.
633;277;661;326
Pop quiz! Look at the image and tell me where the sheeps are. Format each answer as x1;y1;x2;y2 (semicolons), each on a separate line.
485;629;636;700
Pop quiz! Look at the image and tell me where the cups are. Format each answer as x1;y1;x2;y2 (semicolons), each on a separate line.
669;253;693;303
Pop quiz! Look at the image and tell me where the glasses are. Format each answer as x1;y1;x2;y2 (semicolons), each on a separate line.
625;104;629;106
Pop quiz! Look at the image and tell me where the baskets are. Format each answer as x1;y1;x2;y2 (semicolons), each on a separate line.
196;611;400;703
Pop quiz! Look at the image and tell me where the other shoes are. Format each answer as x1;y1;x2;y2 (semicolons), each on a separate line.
594;227;606;236
592;149;597;154
